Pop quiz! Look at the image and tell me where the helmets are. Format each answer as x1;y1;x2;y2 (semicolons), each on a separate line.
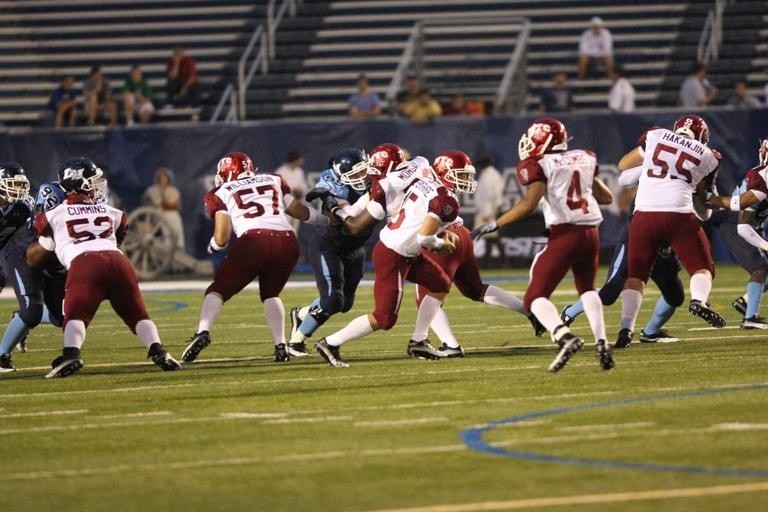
433;150;476;194
366;142;407;176
58;156;107;204
758;138;768;167
214;151;255;187
673;114;710;145
517;117;568;161
332;147;369;192
0;162;31;202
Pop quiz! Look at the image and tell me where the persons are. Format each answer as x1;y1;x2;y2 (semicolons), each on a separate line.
536;72;575;113
390;70;424;115
46;74;77;128
162;45;200;110
1;113;767;380
119;62;154;129
575;16;614;79
603;61;636;113
400;88;443;124
443;94;481;115
81;63;117;127
346;73;382;118
725;79;761;107
674;62;719;109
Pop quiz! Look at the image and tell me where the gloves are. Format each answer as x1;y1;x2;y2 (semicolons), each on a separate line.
306;187;338;210
204;238;228;255
470;221;498;243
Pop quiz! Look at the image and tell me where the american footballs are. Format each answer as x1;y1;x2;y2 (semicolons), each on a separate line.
432;229;460;255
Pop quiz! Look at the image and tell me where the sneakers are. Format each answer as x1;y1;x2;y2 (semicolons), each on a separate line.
639;328;683;343
549;332;585;372
44;354;84;378
313;336;350;368
528;305;577;337
152;349;181;371
616;328;633;348
0;353;15;369
274;307;308;363
689;299;727;328
407;338;465;360
182;329;210;362
594;338;615;369
12;311;28;353
732;296;768;329
